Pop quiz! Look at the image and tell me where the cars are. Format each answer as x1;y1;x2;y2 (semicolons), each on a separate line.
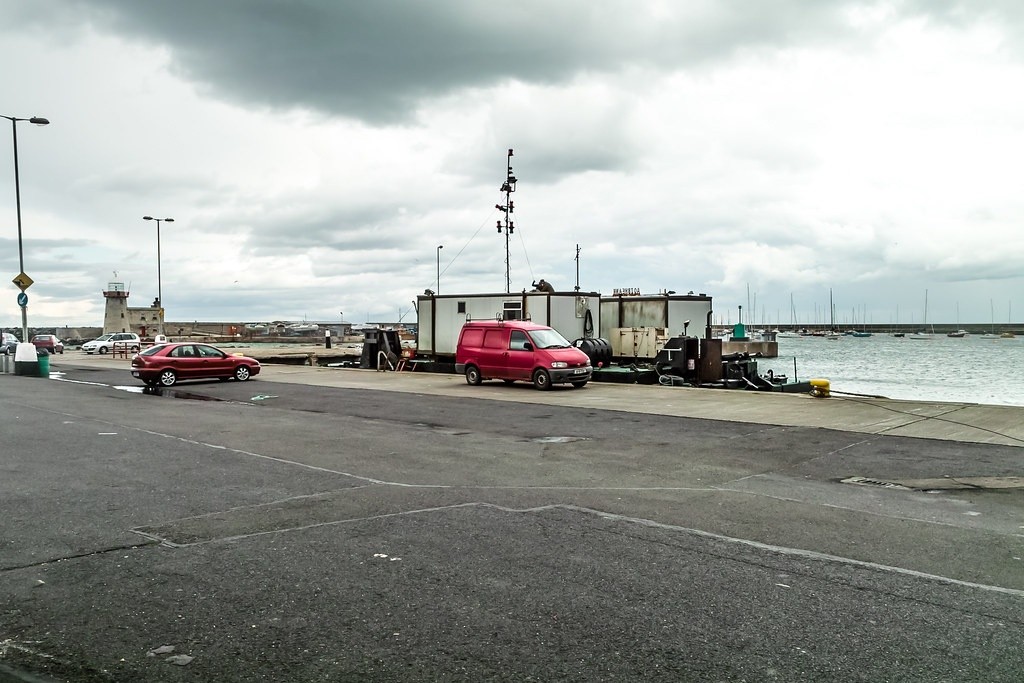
130;341;262;387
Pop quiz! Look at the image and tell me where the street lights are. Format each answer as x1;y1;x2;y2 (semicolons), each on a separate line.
0;113;51;344
340;311;343;343
437;245;444;296
141;216;174;335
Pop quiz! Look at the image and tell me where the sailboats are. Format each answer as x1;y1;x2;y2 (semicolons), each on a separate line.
908;290;936;339
712;283;875;341
890;305;906;338
998;300;1016;338
979;298;1002;340
946;300;970;338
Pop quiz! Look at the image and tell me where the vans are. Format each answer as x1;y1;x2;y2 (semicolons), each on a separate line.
454;310;594;391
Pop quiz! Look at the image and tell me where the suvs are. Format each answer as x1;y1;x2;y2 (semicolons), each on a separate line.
30;334;65;354
80;331;143;354
0;333;22;355
